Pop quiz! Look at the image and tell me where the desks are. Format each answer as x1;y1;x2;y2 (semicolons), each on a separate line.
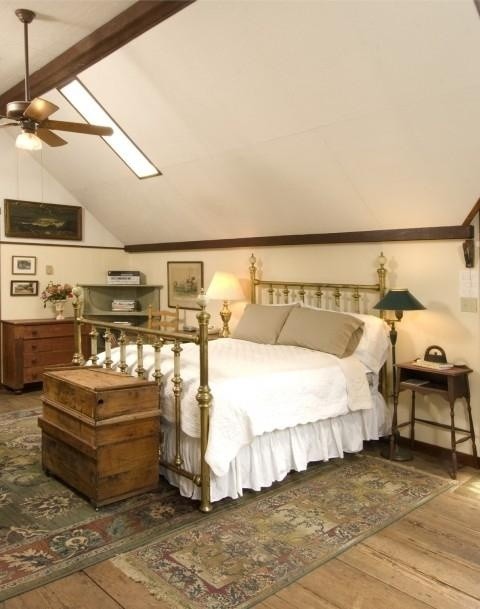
169;327;222;344
387;358;480;479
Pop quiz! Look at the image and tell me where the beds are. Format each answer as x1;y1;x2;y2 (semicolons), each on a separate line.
62;246;392;516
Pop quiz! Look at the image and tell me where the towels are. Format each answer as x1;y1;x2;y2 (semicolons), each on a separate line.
335;354;375;413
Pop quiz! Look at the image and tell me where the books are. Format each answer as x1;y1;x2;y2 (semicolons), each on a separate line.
401;360;453;391
112;299;137;312
113;322;136;326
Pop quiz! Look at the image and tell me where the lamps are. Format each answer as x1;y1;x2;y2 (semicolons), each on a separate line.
372;287;429;463
12;117;46;152
206;270;246;338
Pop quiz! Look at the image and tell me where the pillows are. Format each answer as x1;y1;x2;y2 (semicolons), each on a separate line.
227;301;302;346
296;302;395;376
274;305;366;360
257;300;304;307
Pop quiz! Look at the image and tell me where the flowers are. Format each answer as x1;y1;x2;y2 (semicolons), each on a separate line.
40;279;74;310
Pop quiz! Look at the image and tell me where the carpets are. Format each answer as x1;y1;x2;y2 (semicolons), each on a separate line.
0;395;372;603
109;451;460;609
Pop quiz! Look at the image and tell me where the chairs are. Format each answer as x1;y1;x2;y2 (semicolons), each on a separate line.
146;302;180;346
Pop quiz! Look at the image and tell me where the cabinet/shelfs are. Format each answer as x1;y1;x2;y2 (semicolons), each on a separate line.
0;318;91;395
74;281;165;356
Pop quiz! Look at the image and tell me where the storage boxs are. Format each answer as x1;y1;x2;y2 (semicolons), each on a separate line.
35;362;165;511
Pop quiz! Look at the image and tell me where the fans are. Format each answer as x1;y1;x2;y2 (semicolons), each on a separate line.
0;8;114;151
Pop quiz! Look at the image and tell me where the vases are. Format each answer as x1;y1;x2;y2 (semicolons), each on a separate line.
51;299;68;320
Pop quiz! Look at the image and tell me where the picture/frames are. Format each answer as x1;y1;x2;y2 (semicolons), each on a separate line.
11;279;39;297
12;256;37;275
4;198;82;242
166;260;205;312
